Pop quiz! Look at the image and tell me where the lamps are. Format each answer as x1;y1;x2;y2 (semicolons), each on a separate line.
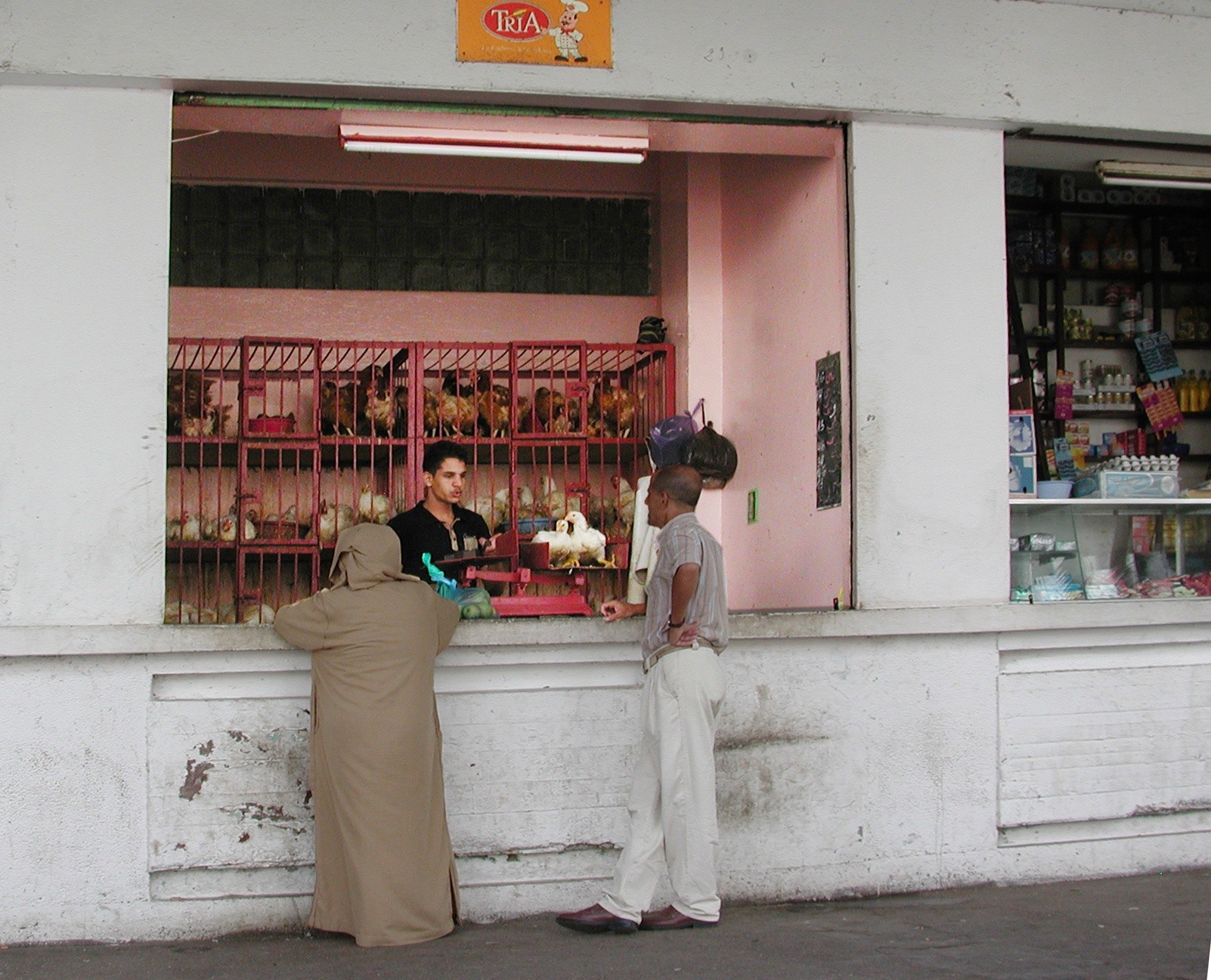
1095;159;1211;189
339;124;649;164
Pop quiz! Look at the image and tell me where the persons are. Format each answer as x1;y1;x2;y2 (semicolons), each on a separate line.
386;439;492;585
272;521;461;947
554;464;730;935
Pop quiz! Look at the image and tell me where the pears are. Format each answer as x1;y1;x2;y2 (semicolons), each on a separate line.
457;592;494;619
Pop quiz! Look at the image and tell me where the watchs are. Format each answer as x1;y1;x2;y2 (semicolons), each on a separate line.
666;614;686;628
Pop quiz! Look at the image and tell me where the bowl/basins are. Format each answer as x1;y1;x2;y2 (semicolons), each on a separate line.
500;518;551;534
253;519;309;539
1037;480;1076;498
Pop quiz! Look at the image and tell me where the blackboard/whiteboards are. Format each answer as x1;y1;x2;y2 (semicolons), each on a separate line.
816;353;842;512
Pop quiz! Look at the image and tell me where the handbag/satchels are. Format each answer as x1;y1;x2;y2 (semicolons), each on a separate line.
645;399;703;469
421;551;496;618
680;399;739;482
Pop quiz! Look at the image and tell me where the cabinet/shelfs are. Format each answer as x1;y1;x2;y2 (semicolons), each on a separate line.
1005;165;1211;498
1010;505;1211;600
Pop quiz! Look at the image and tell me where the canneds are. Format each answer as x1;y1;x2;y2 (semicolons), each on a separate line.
1073;360;1133;403
1103;284;1153;341
1061;318;1094;340
1033;325;1042;336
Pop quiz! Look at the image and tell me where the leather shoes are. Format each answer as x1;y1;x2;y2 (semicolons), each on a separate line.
556;904;637;935
642;905;718;929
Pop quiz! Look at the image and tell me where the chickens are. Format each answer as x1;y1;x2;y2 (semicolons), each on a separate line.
165;473;637;542
530;510;618;569
165;586;275;625
167;367;647;438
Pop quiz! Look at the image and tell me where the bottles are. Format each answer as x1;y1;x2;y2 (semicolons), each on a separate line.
1176;368;1211;411
1071;360;1133;403
1032;325;1043;336
1057;223;1140;272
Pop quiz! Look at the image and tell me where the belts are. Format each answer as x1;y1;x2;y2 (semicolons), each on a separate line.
643;638;719;672
